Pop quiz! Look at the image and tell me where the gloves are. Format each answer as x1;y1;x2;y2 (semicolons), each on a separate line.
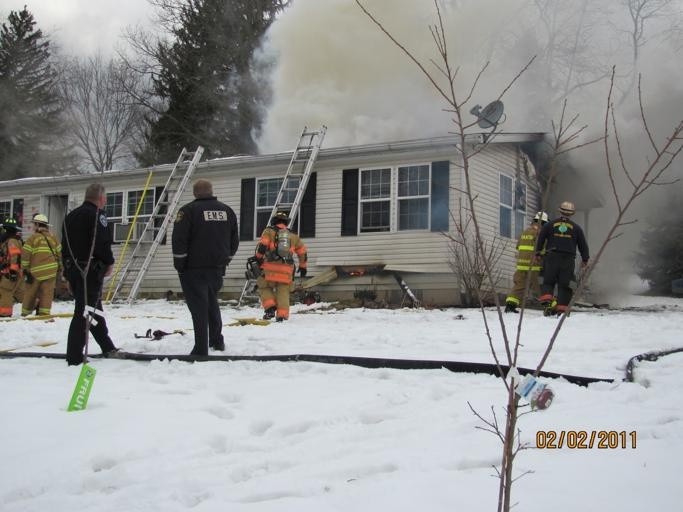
9;269;34;284
297;267;306;277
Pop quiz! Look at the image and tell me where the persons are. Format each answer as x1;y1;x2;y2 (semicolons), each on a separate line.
172;179;238;356
535;200;590;318
61;182;123;365
0;212;64;322
256;211;308;321
504;211;557;313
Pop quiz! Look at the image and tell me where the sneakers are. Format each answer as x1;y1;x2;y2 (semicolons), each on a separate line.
263;308;275;320
540;300;565;317
505;301;519;313
277;318;286;321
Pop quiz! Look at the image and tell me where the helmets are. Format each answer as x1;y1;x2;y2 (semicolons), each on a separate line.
533;212;548;222
2;218;18;228
271;212;291;225
557;201;575;215
24;214;55;227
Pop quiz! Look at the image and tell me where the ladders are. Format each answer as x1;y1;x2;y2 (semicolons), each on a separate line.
238;125;328;307
112;146;203;306
